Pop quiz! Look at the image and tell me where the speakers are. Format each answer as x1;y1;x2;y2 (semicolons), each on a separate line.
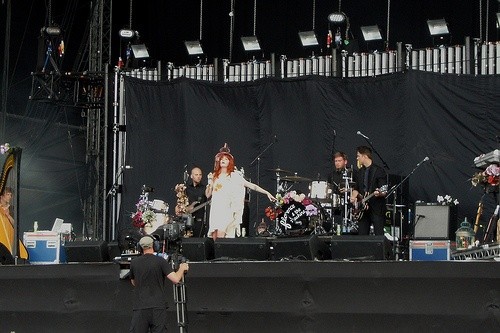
64;240;110;262
414;204;449;239
182;234;395;262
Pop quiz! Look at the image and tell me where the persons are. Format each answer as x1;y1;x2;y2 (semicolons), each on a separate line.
328;152;357;199
185;167;206;238
351;146;387;235
130;237;189;333
205;152;276;241
0;187;15;226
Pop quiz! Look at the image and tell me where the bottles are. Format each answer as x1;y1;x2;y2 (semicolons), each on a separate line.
369;225;376;236
455;217;476;250
34;221;38;232
337;225;341;236
242;227;247;237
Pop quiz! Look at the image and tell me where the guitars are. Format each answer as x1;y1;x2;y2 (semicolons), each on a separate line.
175;200;210;217
353;185;388;221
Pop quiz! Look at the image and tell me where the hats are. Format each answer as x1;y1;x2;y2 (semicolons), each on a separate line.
139;236;154;248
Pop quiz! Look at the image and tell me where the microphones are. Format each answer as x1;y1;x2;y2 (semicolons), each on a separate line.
356;131;368;138
415;157;429;166
416;214;425;219
275;136;279;143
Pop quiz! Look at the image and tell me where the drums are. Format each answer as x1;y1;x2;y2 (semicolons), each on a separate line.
150;198;170;214
310;180;330;202
320;193;340;207
142;212;172;236
275;197;319;237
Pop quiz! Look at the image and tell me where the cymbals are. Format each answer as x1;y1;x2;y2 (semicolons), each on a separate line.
283;176;303;181
265;168;290;173
